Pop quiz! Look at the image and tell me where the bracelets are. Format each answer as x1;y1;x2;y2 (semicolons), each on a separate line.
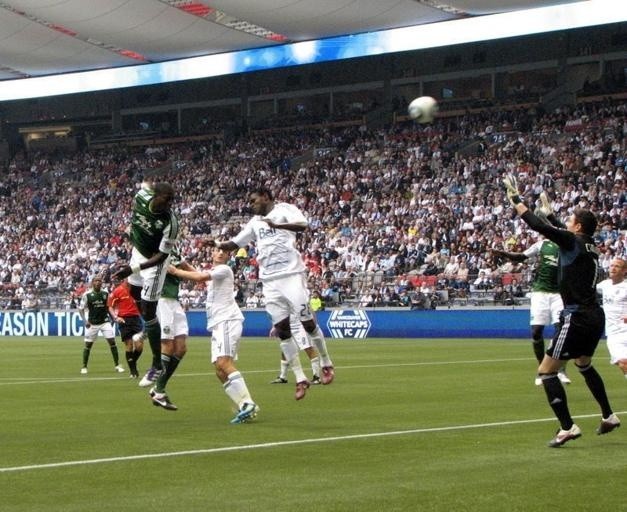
215;239;221;248
129;263;141;274
114;316;118;321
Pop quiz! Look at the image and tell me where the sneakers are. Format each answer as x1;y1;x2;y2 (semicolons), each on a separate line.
270;377;287;383
310;365;335;385
557;373;571;384
596;413;620;435
81;368;88;374
139;368;162;386
548;423;583;447
116;366;125;373
151;390;178;410
230;402;260;423
296;380;310;399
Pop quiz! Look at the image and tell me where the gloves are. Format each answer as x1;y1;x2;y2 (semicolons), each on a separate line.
503;174;522;206
539;193;554;219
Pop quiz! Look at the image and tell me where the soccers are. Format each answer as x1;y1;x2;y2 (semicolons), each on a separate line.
409;96;438;124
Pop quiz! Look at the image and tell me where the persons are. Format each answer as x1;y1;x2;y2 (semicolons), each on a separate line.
108;277;146;379
204;187;334;400
149;247;189;410
267;306;321;384
595;258;627;378
485;232;571;386
78;277;125;373
111;173;179;387
502;172;620;446
166;245;261;424
